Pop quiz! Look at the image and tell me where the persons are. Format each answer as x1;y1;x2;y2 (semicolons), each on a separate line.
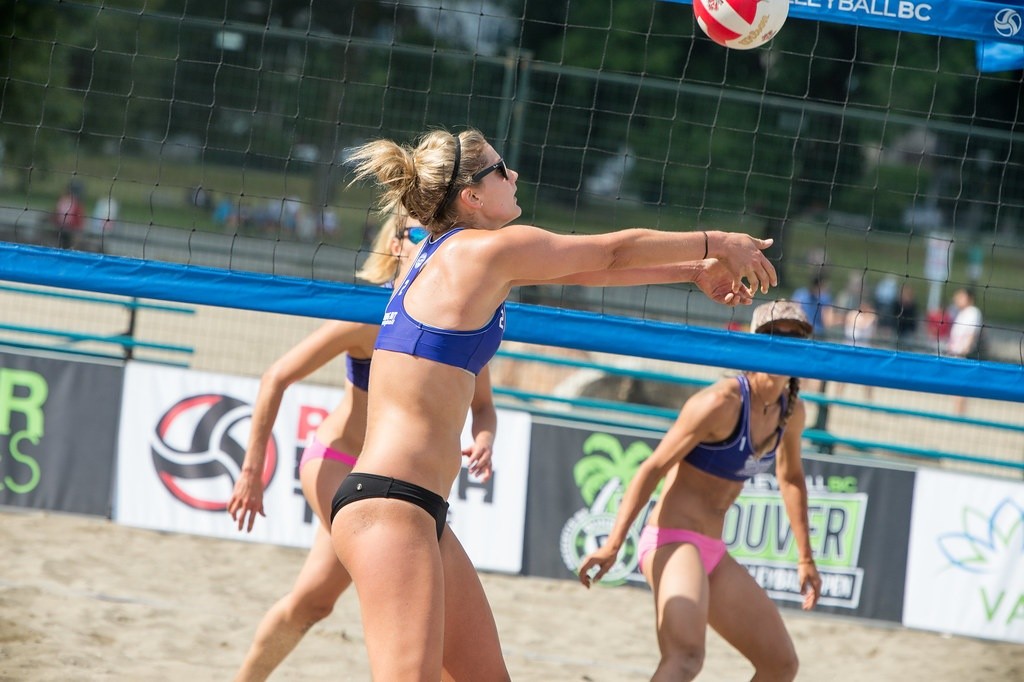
331;131;777;682
227;202;512;682
578;297;823;682
792;270;989;418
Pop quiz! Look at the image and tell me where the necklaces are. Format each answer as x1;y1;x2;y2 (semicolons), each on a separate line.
754;372;777;415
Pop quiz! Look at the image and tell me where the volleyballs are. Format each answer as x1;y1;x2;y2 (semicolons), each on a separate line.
692;0;789;50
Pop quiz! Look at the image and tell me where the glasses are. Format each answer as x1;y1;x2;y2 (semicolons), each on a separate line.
763;329;804;338
467;157;508;182
401;227;430;245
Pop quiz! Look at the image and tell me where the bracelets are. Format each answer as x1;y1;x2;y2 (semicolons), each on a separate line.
798;559;815;564
702;232;709;260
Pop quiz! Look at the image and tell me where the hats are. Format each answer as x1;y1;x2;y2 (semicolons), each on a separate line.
750;297;813;334
